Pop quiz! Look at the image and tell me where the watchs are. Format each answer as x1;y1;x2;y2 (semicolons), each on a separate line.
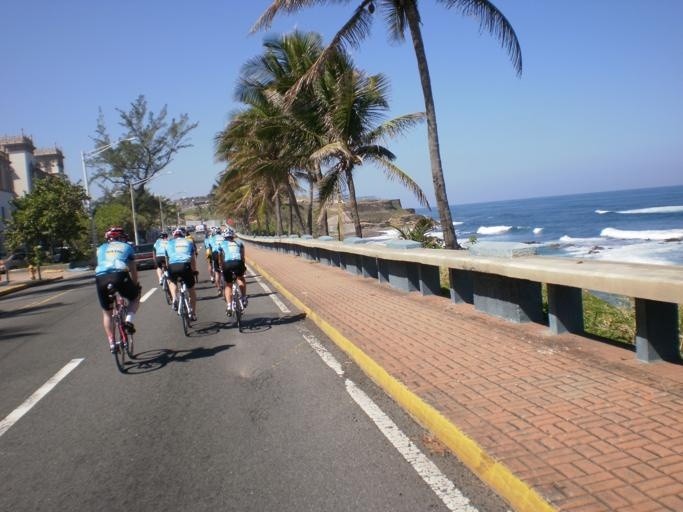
95;227;142;354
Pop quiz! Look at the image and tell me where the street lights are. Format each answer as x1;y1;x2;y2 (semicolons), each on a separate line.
129;169;189;254
79;134;140;250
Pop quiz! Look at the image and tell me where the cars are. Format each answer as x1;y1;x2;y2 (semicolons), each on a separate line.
0;252;34;270
178;224;221;235
131;242;155;269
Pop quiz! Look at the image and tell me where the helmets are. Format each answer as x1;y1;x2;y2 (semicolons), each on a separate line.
213;227;234;238
159;226;189;237
104;226;128;240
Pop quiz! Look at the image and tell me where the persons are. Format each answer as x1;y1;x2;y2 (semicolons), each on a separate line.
152;226;249;321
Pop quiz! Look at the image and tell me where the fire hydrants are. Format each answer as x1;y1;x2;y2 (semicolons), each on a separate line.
28;264;36;280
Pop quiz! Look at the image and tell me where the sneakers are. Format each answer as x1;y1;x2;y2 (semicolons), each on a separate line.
192;313;197;321
218;289;223;296
172;298;178;311
211;276;215;281
243;299;248;306
110;342;120;353
225;307;232;317
123;321;136;334
159;278;164;286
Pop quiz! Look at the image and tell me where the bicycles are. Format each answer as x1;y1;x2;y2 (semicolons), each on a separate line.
158;251;248;338
105;279;142;372
